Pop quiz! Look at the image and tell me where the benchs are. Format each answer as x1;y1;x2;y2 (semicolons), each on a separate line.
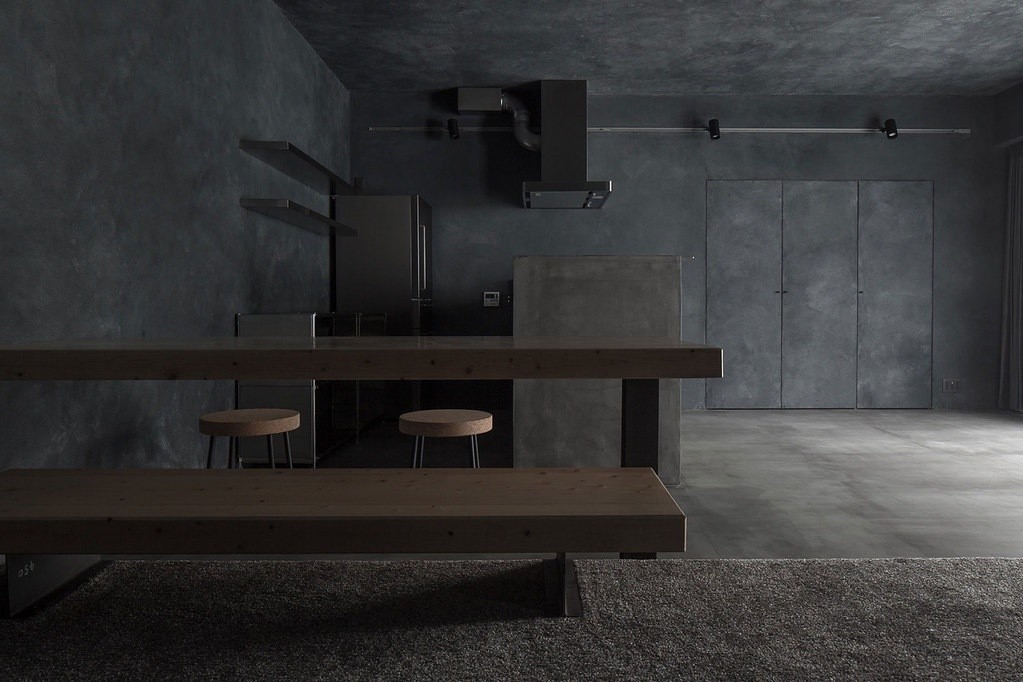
0;467;686;620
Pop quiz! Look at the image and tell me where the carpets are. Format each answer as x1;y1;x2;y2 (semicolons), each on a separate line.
1;556;1023;682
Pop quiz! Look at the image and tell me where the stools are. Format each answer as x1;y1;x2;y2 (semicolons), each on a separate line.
399;409;492;468
199;409;300;469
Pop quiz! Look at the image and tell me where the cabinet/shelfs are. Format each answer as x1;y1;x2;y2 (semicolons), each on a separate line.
514;254;682;486
358;311;398;432
237;311;358;468
239;139;334;237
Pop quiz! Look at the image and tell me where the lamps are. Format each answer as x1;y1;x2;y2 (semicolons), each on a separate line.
448;118;459;139
706;119;720;140
878;118;898;139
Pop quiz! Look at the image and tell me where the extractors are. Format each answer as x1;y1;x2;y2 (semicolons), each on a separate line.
458;80;612;210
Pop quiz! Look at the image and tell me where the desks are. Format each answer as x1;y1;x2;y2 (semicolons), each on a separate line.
0;337;724;560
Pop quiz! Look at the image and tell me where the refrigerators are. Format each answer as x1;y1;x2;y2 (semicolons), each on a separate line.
333;191;434;423
